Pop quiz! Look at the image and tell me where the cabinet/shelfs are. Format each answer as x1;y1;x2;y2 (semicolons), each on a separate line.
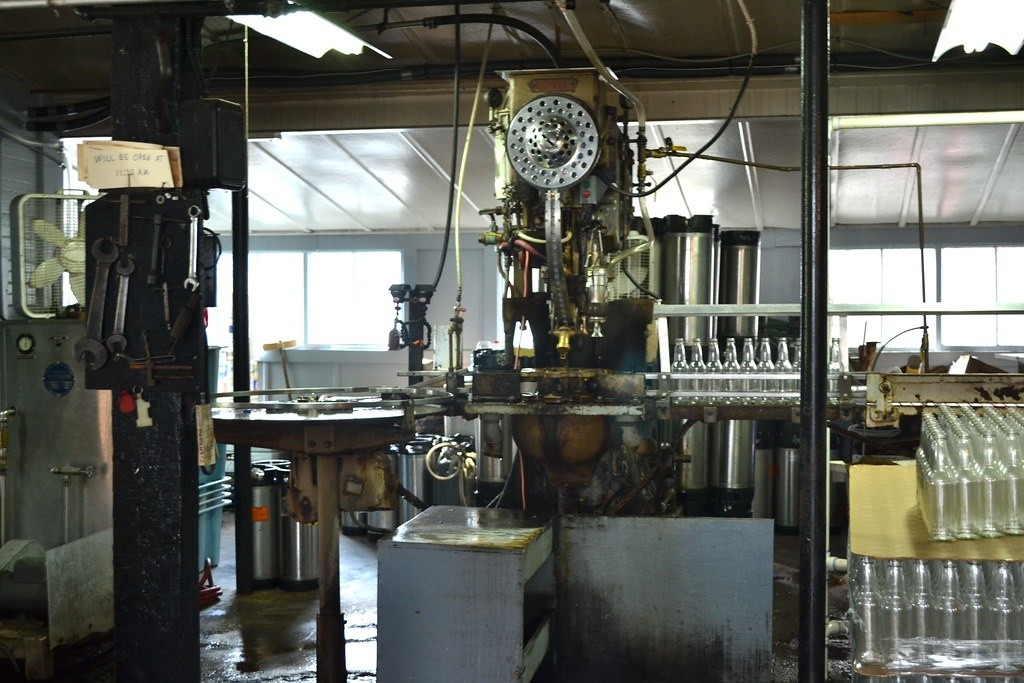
375;506;555;683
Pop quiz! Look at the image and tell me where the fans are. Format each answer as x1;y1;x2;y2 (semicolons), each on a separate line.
11;192;106;318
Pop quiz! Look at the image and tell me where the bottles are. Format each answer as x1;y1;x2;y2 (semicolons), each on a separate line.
845;553;1024;682
755;337;775;406
687;338;706;405
669;337;689;405
827;336;846;401
737;338;757;406
791;338;803;404
720;338;740;406
705;337;723;406
775;337;794;405
914;405;1023;540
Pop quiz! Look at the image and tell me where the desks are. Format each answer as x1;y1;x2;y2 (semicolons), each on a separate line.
847;464;1022;682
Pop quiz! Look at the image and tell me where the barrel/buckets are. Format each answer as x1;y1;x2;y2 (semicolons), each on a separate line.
248;460;320;590
337;435;475;536
661;419;846;532
610;208;760;366
